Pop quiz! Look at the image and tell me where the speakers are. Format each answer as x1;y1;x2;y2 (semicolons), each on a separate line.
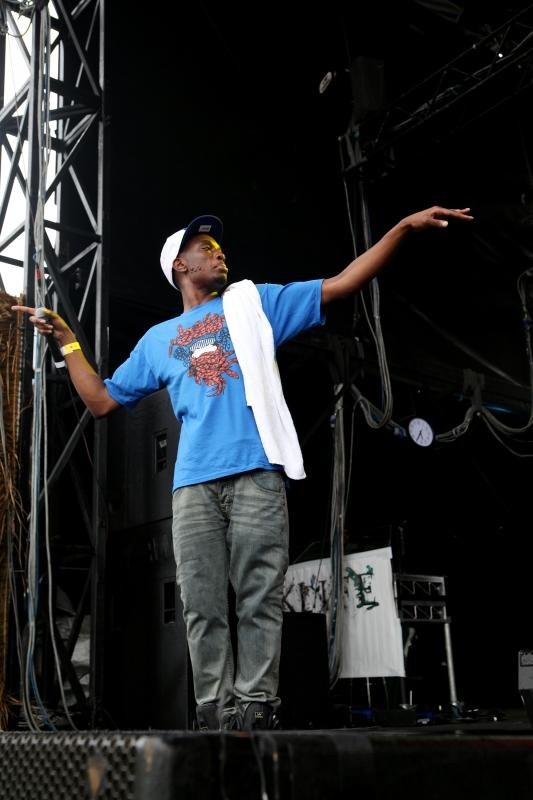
102;519;195;730
109;388;179;525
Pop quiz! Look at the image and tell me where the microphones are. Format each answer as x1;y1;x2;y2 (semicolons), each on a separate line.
36;307;67;376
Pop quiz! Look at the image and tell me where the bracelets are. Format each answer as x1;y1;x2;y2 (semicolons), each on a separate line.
59;342;81;357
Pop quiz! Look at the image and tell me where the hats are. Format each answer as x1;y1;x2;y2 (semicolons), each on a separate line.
160;215;222;290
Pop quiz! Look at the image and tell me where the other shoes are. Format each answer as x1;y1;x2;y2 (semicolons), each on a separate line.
196;702;227;730
238;702;280;731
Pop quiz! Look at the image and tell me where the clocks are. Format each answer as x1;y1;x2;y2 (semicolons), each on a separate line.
408;418;433;446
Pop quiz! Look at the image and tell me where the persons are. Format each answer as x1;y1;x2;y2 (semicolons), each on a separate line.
11;206;475;732
